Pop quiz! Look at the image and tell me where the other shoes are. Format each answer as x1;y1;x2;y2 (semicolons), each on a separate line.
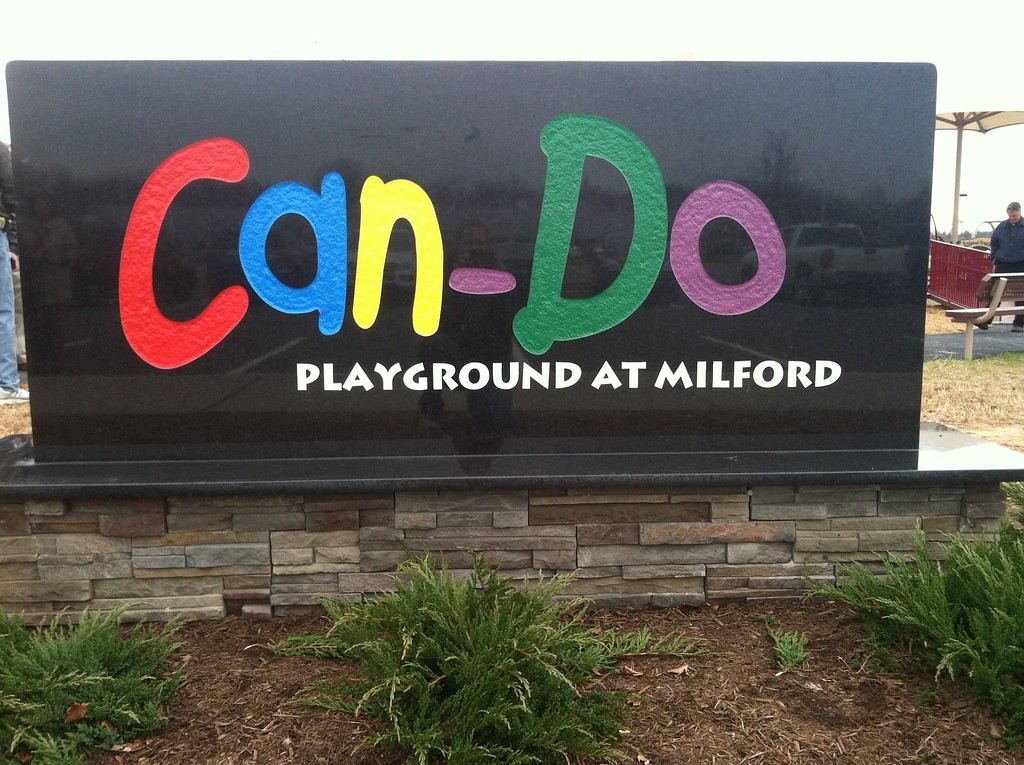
974;322;988;329
1011;326;1023;332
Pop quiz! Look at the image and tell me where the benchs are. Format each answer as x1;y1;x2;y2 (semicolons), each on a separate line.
944;272;1024;360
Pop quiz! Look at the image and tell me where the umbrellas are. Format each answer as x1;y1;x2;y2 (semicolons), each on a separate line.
935;111;1024;245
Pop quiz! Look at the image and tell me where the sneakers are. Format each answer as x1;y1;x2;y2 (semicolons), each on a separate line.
0;385;30;405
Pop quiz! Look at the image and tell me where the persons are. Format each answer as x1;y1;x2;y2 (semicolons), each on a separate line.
970;201;1024;334
0;142;29;400
